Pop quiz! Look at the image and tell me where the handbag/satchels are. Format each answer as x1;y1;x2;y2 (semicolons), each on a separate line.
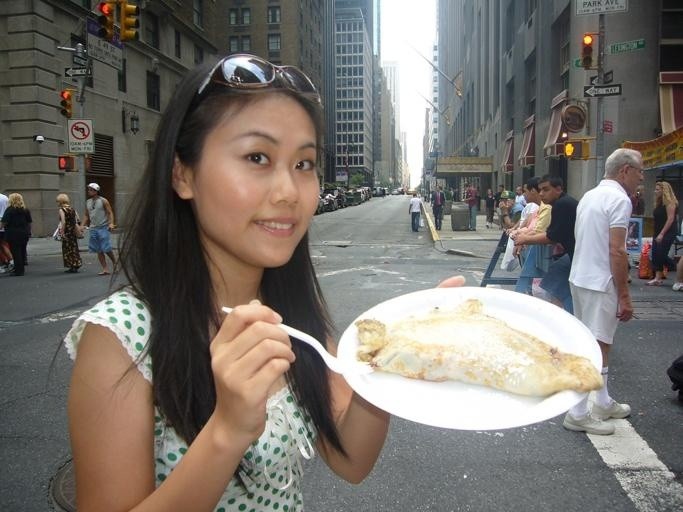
74;224;84;238
53;225;63;241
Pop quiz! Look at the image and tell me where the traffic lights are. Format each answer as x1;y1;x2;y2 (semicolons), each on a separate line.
564;141;589;160
118;0;140;43
97;0;116;42
59;88;73;118
581;34;593;71
59;157;74;169
87;158;95;171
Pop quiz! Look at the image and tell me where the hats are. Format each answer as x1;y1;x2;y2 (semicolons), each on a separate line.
500;190;515;198
87;183;100;191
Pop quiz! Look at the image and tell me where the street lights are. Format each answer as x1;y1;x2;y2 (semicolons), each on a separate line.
433;141;441;190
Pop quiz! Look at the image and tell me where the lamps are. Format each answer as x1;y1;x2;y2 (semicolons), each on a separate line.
122;107;139;135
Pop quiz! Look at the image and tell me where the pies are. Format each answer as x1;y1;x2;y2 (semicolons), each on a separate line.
356;298;605;402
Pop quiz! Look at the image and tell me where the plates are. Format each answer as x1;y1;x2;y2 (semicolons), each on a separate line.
336;285;603;432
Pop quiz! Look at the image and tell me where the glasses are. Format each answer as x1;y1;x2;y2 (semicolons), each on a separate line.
188;54;321;112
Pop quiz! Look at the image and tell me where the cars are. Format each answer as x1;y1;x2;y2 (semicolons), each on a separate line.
313;186;390;215
390;187;417;195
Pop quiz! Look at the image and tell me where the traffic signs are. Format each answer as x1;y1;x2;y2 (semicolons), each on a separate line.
608;38;647;56
73;56;94;68
584;85;622;97
63;66;92;78
590;70;614;86
574;58;597;68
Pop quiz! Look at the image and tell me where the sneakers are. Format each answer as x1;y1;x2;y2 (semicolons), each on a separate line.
672;282;683;291
64;267;80;273
646;278;664;286
563;409;615;434
592;397;631;420
98;263;119;275
0;258;28;276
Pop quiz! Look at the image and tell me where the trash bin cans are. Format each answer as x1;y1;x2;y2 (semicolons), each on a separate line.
451;202;471;231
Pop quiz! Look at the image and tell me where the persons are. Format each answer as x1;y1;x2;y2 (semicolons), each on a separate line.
562;148;644;436
56;193;82;273
79;183;120;275
430;174;683;316
62;54;467;512
0;192;33;276
408;192;423;232
